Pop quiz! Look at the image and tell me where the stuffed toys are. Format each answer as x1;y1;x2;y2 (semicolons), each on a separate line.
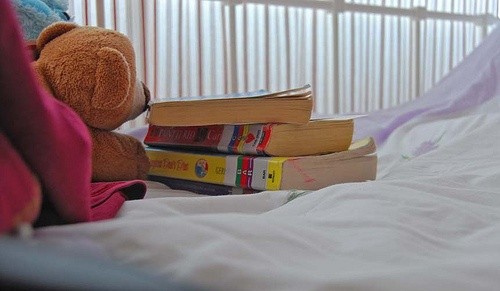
28;21;151;181
11;0;75;41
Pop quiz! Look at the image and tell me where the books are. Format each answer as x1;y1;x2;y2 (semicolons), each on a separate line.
144;135;378;191
143;117;356;157
145;83;313;128
